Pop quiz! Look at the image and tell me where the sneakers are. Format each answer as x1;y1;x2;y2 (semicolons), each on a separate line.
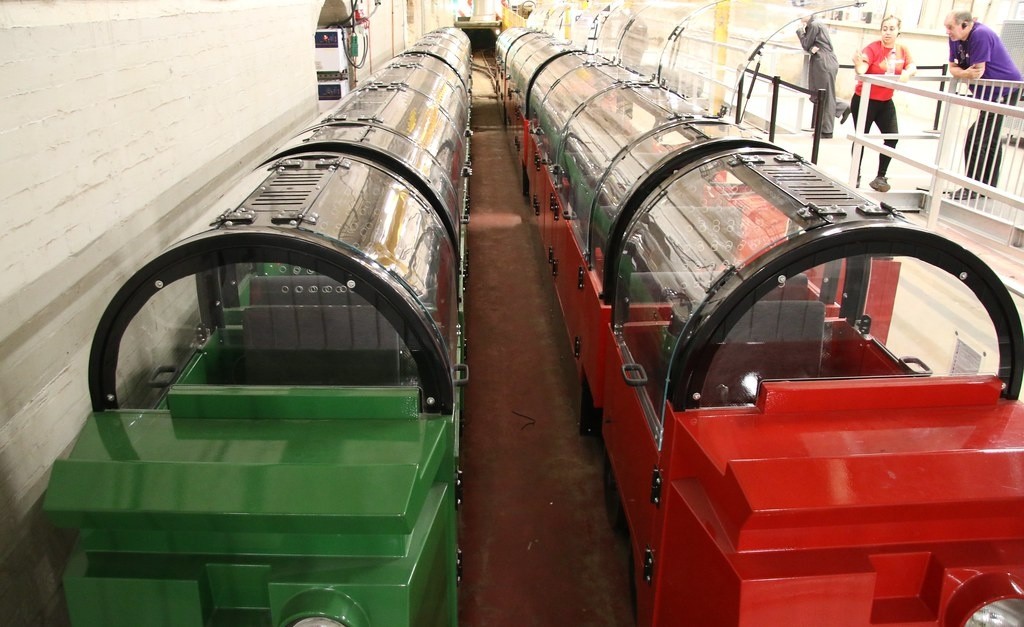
869;176;890;192
950;187;978;199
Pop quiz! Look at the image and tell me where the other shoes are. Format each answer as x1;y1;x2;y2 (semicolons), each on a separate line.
840;107;851;124
813;132;833;138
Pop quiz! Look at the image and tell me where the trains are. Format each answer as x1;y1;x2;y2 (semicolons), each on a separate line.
496;25;1023;626
42;27;473;627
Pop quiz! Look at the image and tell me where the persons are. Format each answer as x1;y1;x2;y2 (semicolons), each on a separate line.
851;14;917;193
943;7;1023;200
795;15;851;140
